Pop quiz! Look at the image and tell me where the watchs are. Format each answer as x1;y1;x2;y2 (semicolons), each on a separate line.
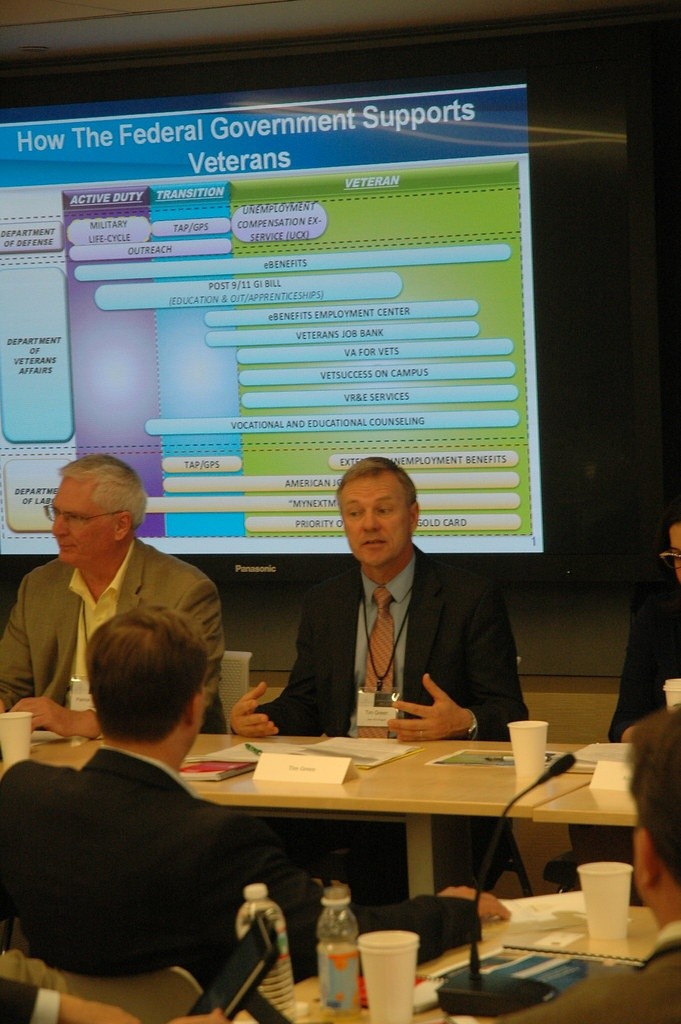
466;709;476;739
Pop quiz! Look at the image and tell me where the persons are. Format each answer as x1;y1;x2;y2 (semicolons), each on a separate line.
235;457;527;904
492;705;680;1024
0;453;229;740
608;505;681;741
0;607;512;1024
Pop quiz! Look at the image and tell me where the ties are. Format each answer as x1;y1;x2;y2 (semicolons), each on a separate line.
355;587;395;738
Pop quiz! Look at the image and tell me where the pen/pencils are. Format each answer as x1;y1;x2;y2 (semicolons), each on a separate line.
245;744;262;755
484;756;514;761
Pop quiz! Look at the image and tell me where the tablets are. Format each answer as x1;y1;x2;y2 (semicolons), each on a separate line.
186;914;278;1020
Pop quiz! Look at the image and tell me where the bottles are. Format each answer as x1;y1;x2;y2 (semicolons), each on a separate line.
234;882;296;1023
316;884;362;1024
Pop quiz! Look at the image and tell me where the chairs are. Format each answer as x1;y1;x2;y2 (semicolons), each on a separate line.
0;950;202;1024
474;655;535;898
216;650;253;733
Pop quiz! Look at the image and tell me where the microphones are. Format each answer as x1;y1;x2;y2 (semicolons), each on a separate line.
435;753;577;1016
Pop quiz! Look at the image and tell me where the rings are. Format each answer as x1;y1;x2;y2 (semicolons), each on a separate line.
420;731;422;737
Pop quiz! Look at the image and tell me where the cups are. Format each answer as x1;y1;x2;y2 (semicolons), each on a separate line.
662;678;680;711
0;712;32;771
358;930;420;1024
506;721;549;773
577;861;633;941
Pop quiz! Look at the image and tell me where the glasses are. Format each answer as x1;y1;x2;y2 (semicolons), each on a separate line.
659;548;681;568
43;503;126;529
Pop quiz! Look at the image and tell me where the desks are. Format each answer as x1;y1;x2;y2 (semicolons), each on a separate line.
0;730;640;900
232;889;659;1024
